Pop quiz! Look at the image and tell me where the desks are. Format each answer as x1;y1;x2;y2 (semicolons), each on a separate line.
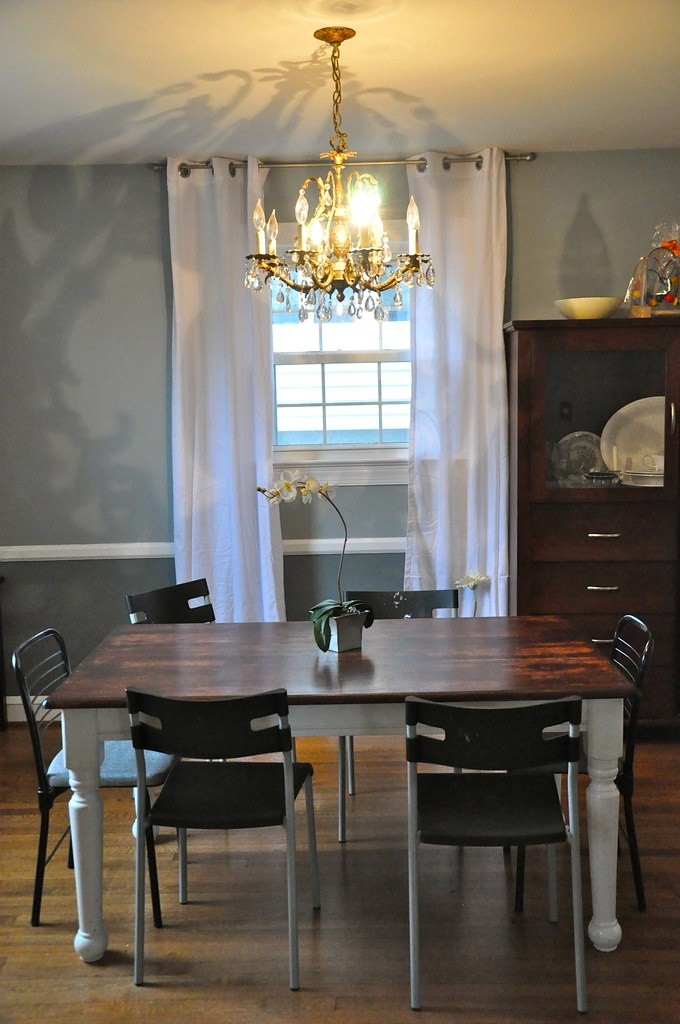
43;620;632;966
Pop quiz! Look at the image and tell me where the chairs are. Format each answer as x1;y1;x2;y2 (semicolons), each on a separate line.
121;578;295;764
399;694;588;1019
337;584;464;844
13;623;185;926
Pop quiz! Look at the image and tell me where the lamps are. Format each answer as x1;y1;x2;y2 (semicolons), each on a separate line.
241;26;436;322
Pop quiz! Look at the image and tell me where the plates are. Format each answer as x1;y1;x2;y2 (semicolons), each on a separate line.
599;397;674;488
551;431;612;489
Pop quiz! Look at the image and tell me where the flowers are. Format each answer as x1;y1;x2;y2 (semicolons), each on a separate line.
256;470;376;651
454;571;490;617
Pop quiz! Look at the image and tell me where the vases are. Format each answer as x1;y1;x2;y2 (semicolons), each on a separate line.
309;610;367;652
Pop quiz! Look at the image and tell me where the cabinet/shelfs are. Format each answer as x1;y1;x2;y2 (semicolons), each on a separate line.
500;315;680;741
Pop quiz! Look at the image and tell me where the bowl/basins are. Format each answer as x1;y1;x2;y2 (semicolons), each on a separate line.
554;296;623;320
